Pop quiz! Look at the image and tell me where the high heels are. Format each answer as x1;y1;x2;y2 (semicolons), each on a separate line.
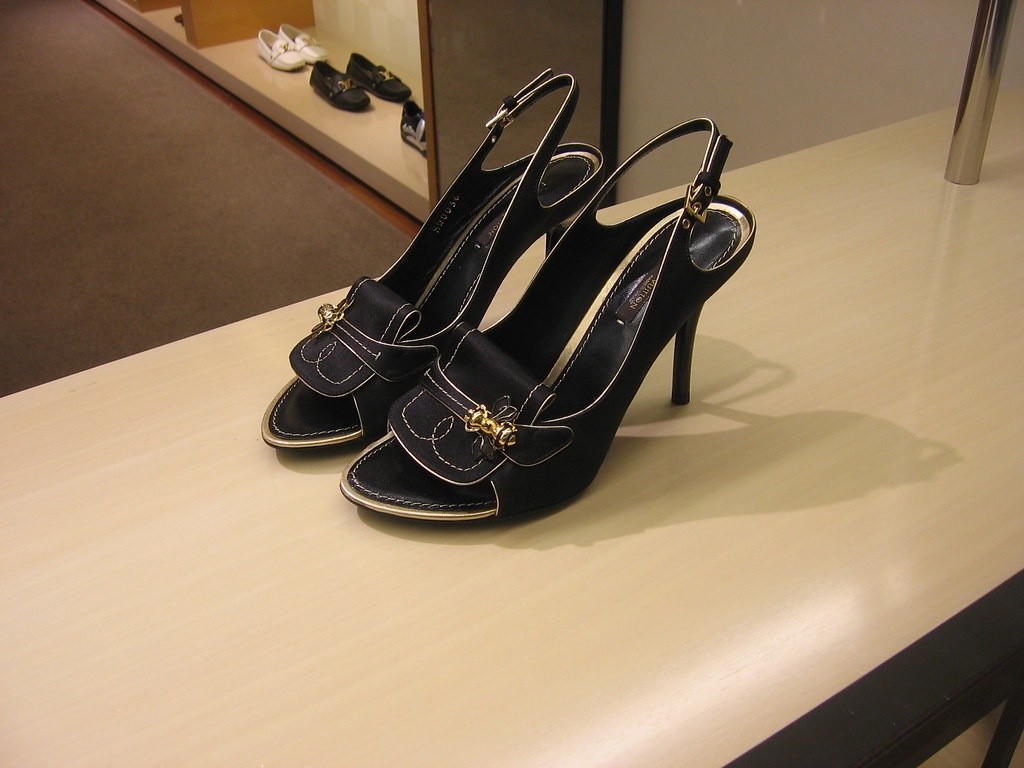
337;116;759;521
260;69;607;448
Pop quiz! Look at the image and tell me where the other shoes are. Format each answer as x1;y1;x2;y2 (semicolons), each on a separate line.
309;61;371;112
400;98;429;158
258;27;306;71
345;52;413;102
279;22;331;64
